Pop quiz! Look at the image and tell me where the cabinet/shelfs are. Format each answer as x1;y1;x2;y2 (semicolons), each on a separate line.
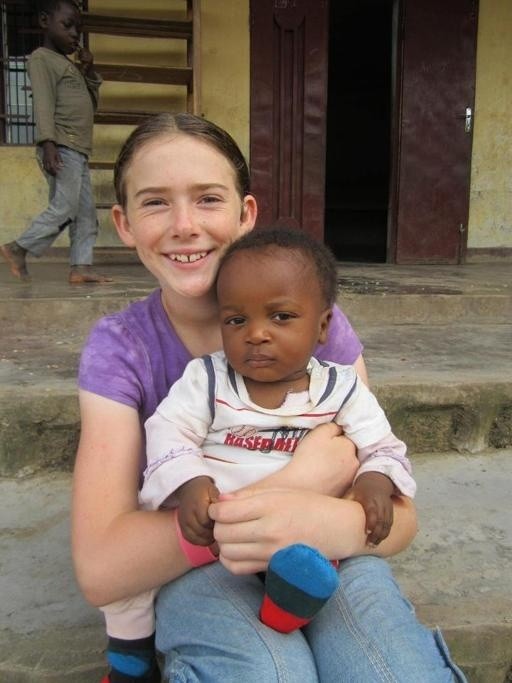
66;0;201;260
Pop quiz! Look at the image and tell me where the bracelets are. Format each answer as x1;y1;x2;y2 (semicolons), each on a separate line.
175;506;218;569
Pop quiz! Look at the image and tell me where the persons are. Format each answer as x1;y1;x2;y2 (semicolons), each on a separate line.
72;112;471;683
96;227;415;682
0;0;113;283
